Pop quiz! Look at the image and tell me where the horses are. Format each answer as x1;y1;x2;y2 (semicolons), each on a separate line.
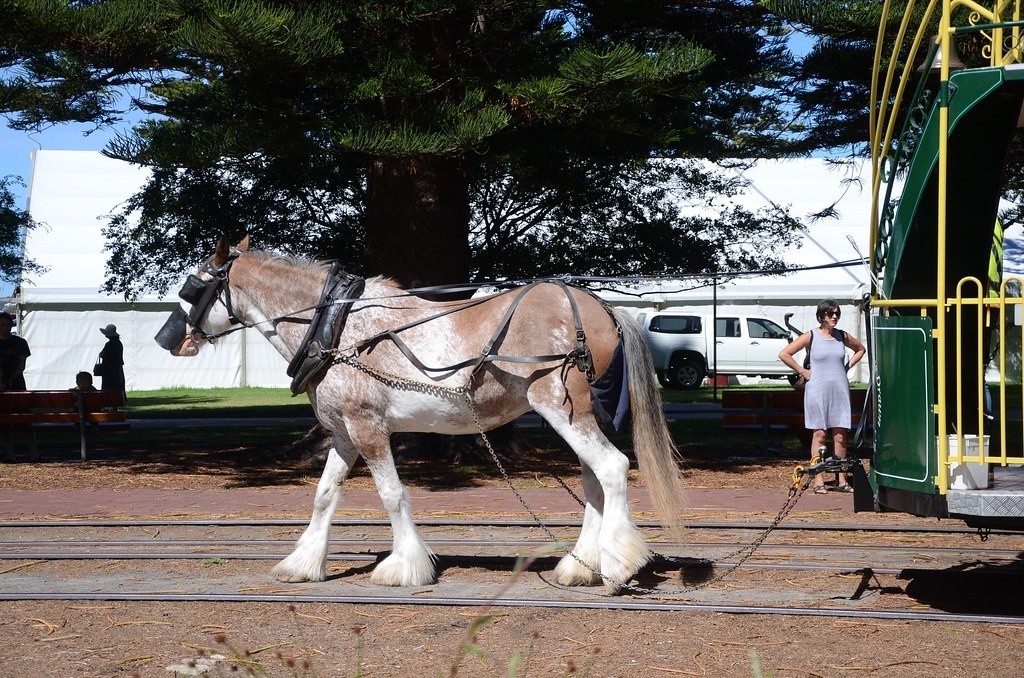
155;235;699;595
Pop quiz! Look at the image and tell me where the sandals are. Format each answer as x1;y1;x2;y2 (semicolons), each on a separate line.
834;482;854;493
814;482;827;494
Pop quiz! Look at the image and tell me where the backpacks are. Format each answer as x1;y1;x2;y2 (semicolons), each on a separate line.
803;329;844;382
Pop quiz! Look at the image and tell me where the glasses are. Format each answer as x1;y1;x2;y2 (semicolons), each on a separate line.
822;311;840;317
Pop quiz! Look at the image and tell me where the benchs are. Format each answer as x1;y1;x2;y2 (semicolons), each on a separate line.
0;390;132;464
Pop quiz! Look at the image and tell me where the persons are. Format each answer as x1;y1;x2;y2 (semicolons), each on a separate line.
99;325;126;408
72;371;98;391
778;299;866;494
0;312;37;461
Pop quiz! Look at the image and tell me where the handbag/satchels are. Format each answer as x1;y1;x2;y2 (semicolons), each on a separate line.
93;353;105;376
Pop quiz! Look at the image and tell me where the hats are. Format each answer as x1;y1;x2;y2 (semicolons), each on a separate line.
100;324;120;336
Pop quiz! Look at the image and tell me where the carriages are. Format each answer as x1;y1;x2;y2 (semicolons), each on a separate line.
154;0;1022;601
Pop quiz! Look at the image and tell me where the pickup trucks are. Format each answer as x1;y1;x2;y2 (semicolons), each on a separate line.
635;312;850;391
